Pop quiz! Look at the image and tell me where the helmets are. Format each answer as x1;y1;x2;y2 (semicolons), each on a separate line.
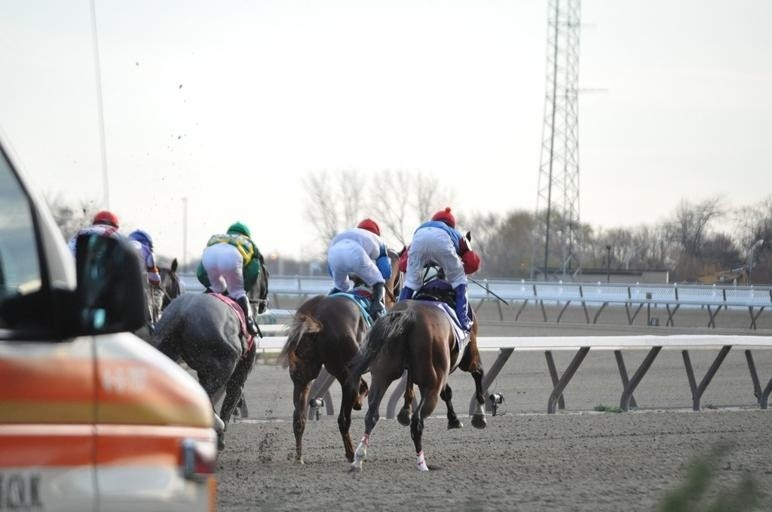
431;207;457;228
128;229;154;248
228;221;251;238
92;210;120;227
357;218;380;236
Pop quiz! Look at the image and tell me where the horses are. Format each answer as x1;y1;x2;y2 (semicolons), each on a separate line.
109;258;183;343
152;258;270;450
282;246;408;465
350;231;487;474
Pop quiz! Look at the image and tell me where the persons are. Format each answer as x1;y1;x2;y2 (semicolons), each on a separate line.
66;210;120;256
399;207;481;329
128;230;162;288
196;221;264;337
326;219;391;319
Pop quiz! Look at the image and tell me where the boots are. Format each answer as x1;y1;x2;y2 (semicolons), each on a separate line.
398;286;413;302
327;287;341;296
203;287;214;295
236;296;259;336
371;282;386;316
453;284;476;331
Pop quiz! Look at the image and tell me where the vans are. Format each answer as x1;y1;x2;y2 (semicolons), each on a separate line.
0;131;223;512
